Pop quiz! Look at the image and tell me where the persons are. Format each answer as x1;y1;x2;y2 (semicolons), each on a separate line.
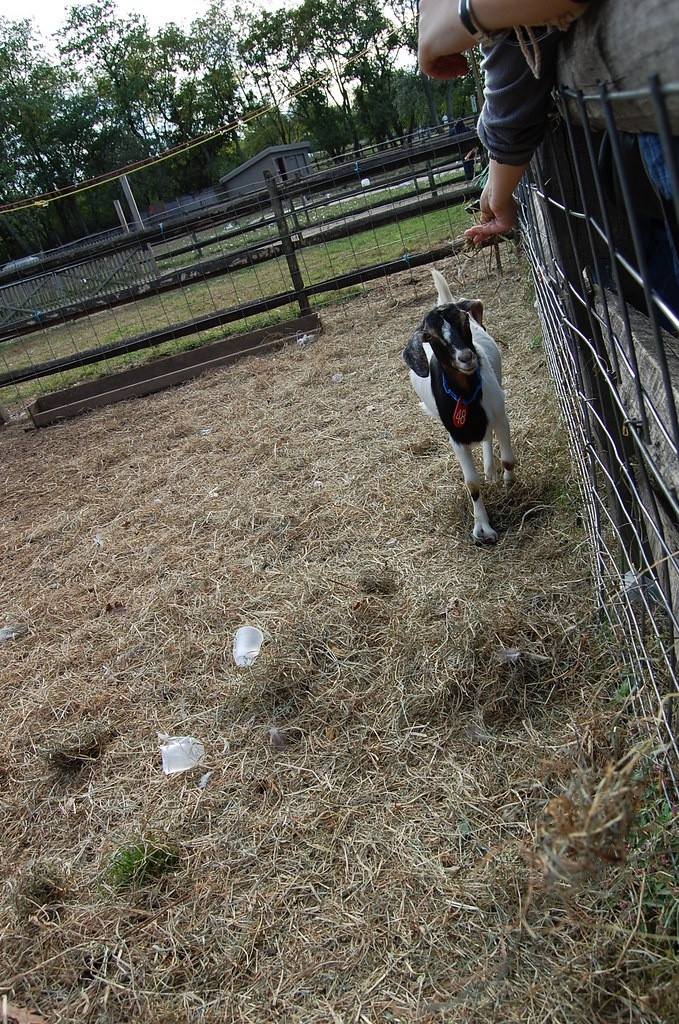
415;112;472;144
411;0;678;353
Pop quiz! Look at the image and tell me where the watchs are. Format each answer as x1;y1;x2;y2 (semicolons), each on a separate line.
457;0;506;47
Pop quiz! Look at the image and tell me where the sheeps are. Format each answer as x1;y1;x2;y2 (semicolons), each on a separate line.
403;268;514;544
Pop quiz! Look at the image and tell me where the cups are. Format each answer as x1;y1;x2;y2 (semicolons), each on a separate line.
162;740;206;775
232;626;264;667
